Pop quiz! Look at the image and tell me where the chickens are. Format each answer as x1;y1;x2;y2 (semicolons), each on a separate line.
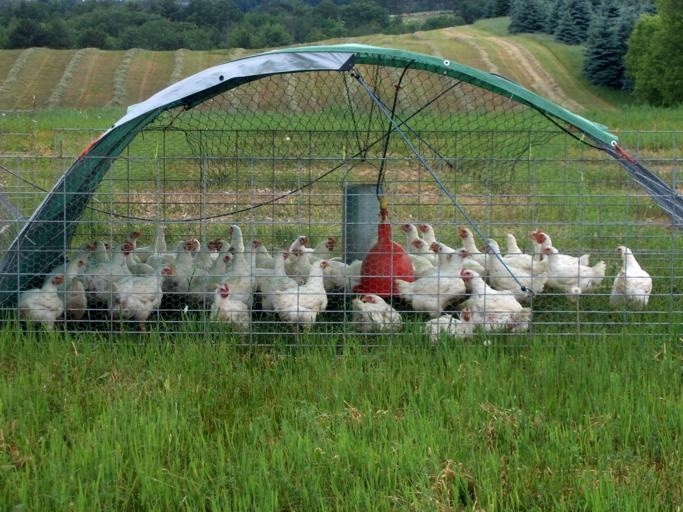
18;222;607;345
607;245;653;315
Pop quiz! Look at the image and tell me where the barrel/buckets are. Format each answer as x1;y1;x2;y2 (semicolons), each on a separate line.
340;161;386;262
